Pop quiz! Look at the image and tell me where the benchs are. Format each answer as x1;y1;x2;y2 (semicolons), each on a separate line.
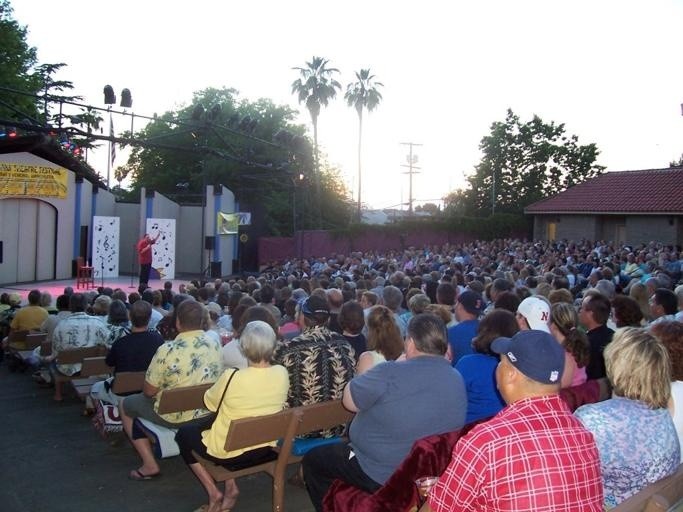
317;380;608;511
16;333;49;370
135;380;213;453
3;331;26;357
190;400;356;512
607;465;680;511
97;371;148;432
72;355;108;397
35;343;53;381
50;345;105;401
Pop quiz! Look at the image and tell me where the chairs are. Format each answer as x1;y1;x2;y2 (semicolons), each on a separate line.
77;256;95;290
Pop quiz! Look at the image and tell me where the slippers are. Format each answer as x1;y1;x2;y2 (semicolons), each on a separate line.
194;503;230;512
128;469;161;481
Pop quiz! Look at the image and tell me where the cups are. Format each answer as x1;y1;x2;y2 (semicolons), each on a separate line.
415;477;440;505
219;332;234;346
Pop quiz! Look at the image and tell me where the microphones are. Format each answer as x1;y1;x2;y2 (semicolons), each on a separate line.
99;255;104;260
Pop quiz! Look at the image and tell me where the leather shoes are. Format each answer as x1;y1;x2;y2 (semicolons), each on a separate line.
80;407;95;416
288;473;308;489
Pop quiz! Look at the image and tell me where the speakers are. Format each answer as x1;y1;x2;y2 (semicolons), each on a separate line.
232;259;239;274
205;236;216;249
211;261;221;278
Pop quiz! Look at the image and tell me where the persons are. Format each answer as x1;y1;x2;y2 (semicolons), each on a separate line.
137;231;161;288
0;236;683;512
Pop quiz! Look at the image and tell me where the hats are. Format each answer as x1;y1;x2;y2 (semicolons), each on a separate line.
459;291;486;316
296;294;330;314
517;296;552;334
490;329;566;384
